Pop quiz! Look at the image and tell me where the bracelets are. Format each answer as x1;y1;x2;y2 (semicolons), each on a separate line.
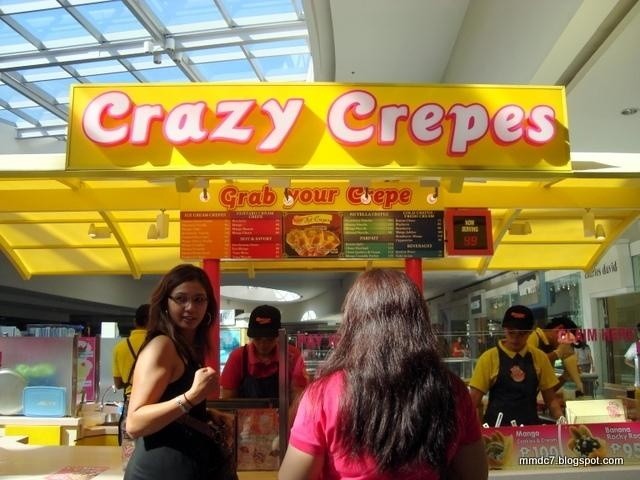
183;393;195;408
175;395;189;414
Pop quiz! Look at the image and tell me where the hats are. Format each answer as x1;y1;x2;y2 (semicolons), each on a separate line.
502;304;535;328
553;318;582;348
247;306;282;339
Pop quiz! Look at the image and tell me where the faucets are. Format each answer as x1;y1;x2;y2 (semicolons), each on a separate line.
101;385;116;406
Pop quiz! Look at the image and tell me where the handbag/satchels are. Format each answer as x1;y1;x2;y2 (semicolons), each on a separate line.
206;421;239;479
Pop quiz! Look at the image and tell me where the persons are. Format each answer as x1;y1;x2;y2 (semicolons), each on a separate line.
468;303;565;427
277;267;489;480
528;316;583;393
219;305;310;401
110;302;152;447
573;333;596;373
623;323;640;421
123;264;239;480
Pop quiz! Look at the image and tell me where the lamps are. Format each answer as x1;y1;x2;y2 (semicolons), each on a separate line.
427;188;439;204
360;187;371;204
200;188;209;202
147;207;169;240
87;223;114;239
549;279;576;292
282;189;294;207
176;178;209;192
508;221;533;234
583;208;606;241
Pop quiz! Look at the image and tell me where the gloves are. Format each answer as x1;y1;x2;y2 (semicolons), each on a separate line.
554;343;573;358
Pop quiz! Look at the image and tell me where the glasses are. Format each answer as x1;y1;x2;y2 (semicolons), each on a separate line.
167;293;211;306
